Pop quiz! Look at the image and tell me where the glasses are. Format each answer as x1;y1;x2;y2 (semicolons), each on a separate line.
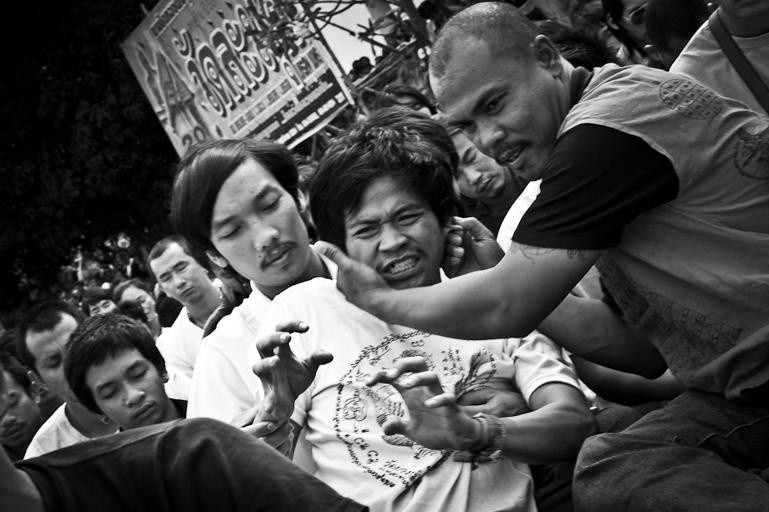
619;4;651;28
